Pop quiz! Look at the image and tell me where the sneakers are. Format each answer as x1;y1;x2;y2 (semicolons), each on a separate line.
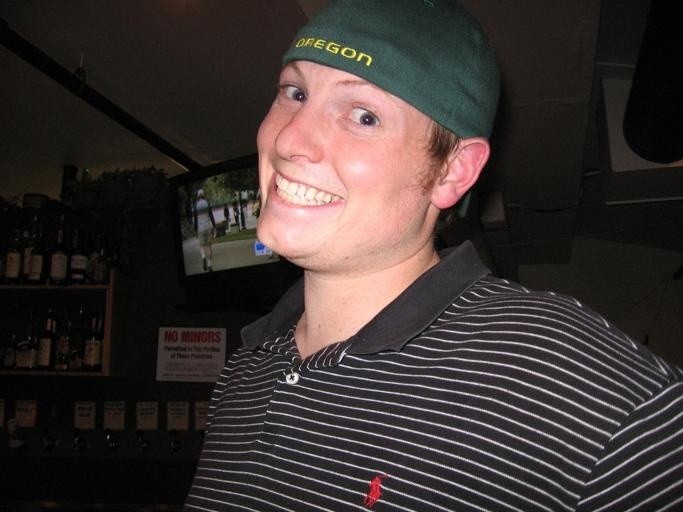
202;256;213;273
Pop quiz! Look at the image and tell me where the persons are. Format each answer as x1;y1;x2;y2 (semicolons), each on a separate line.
182;0;683;511
222;192;261;230
193;188;216;272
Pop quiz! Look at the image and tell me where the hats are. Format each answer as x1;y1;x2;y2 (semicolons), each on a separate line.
197;188;205;198
282;0;502;142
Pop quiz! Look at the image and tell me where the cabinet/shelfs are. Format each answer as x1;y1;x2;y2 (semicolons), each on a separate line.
0;267;117;378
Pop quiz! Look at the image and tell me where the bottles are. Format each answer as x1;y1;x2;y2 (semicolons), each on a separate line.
0;210;108;373
